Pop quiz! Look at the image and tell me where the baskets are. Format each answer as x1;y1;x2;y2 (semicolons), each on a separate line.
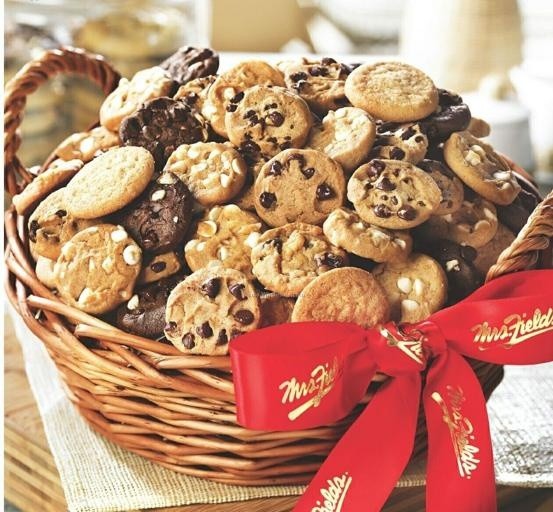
4;45;552;487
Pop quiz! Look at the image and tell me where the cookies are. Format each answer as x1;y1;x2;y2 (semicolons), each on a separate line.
13;45;542;357
